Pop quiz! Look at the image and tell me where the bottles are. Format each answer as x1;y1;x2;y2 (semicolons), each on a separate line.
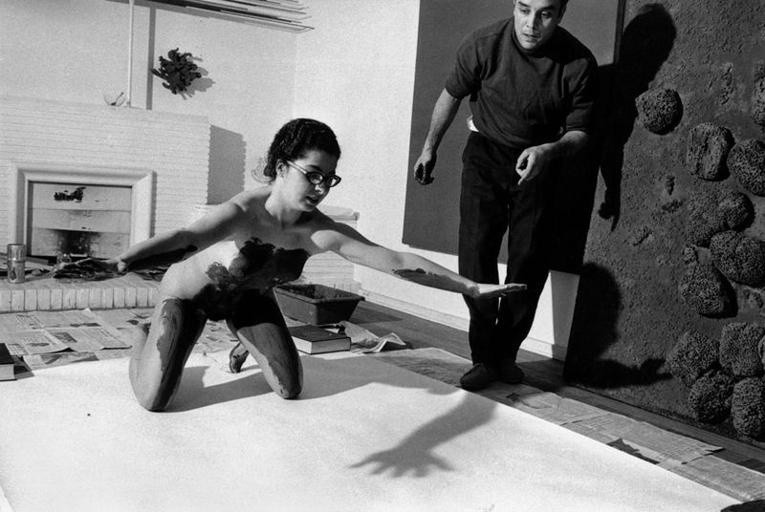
7;244;27;284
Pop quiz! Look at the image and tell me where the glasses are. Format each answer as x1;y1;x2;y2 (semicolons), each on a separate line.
289;161;340;186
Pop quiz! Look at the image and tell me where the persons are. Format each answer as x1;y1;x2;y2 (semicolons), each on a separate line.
413;0;602;393
49;117;532;410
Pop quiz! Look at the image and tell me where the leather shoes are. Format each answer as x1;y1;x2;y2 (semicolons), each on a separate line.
498;362;524;385
461;363;496;390
